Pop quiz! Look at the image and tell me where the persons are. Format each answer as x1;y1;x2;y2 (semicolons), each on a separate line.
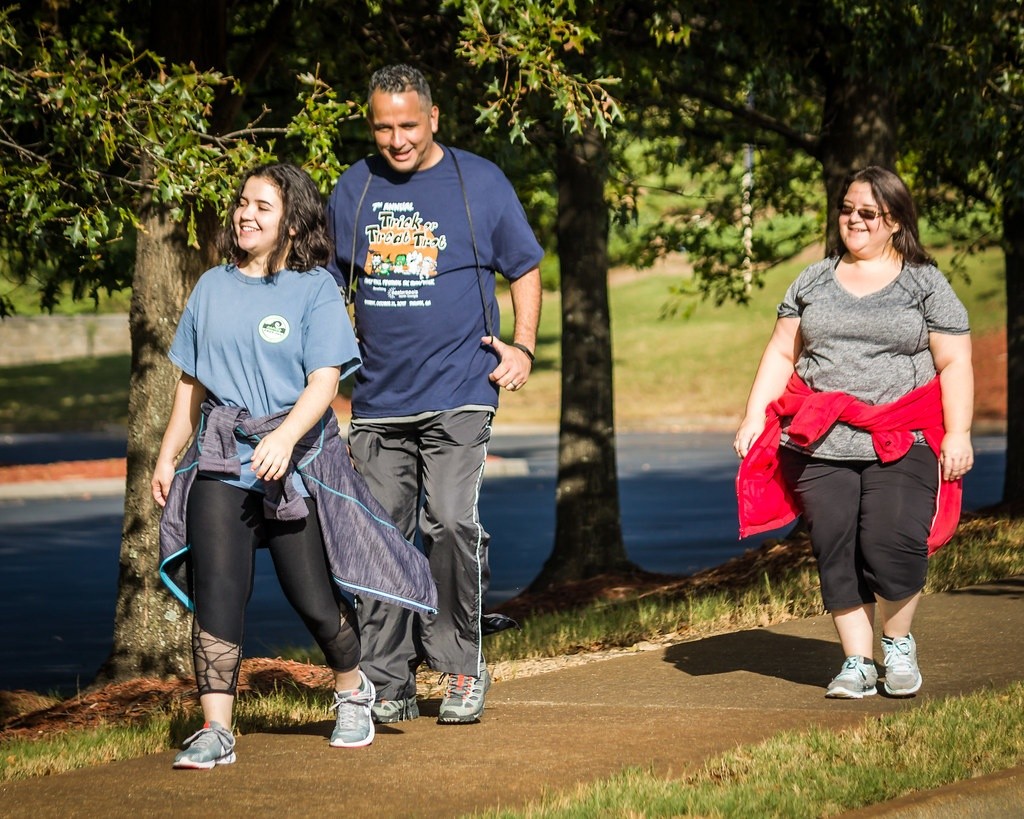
150;159;378;772
321;61;547;727
732;164;975;700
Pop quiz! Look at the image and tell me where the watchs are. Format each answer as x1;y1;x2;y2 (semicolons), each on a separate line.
511;343;536;364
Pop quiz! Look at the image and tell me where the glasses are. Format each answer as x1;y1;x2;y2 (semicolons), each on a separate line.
837;205;891;220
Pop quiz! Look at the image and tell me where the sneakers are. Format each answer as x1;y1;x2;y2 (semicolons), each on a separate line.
436;652;490;722
330;670;377;747
826;659;880;698
173;721;237;770
879;629;924;696
372;694;423;724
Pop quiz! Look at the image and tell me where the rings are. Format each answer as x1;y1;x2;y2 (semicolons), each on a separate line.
510;381;516;388
